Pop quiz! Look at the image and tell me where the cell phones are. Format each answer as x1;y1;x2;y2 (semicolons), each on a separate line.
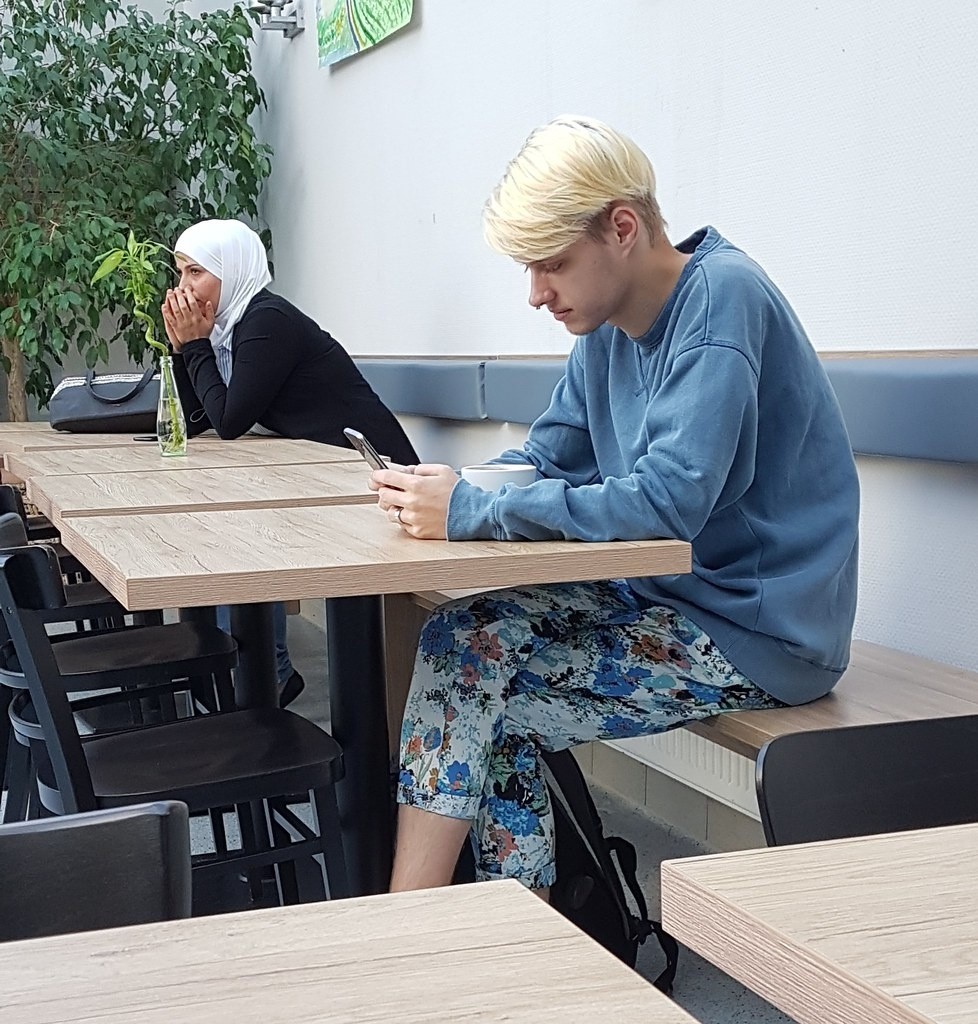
133;436;158;441
343;427;389;472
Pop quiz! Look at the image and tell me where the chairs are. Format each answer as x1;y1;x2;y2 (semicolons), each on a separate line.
1;481;355;944
753;714;978;855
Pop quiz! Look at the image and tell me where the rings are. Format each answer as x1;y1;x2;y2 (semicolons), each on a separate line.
395;507;404;525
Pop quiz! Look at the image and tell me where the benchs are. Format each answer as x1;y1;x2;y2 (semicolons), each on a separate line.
345;350;978;764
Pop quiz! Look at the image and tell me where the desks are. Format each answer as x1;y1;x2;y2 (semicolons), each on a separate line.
1;421;691;914
660;822;978;1024
0;877;702;1024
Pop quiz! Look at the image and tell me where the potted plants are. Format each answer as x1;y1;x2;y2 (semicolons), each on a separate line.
90;231;187;456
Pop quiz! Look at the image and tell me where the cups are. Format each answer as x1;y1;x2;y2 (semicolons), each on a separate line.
461;464;537;494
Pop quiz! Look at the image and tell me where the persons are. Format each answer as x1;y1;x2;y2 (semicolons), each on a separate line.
366;117;860;894
162;219;422;709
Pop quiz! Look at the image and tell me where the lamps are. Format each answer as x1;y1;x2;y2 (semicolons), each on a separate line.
248;0;305;38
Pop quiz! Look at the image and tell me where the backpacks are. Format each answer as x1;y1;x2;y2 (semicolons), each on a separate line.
534;745;679;995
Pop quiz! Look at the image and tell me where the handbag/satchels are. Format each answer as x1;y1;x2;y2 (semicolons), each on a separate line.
47;367;161;434
